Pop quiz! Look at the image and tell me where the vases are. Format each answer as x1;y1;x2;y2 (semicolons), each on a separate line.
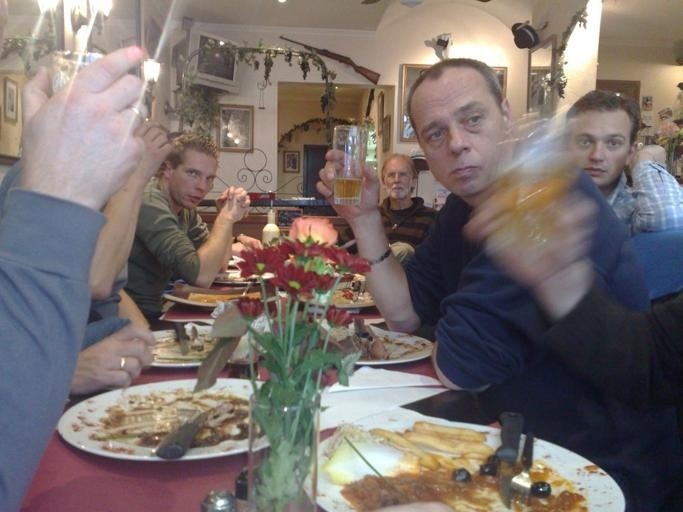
245;386;318;510
664;149;677;176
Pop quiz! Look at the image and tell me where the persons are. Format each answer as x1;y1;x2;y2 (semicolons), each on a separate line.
315;57;683;512
2;45;250;512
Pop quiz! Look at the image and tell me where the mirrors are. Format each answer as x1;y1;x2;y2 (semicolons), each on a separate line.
527;35;557;121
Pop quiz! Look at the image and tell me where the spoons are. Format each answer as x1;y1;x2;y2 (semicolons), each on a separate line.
189;326;203;353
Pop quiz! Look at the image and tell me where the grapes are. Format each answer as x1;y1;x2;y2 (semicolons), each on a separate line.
532;482;551;496
452;463;496;480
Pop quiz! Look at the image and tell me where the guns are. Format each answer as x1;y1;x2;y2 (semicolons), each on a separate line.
280;36;380;86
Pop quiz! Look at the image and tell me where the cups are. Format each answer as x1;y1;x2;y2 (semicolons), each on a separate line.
48;46;104;97
332;125;367;206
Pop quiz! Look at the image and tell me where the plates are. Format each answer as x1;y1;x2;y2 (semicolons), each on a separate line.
210;256;277;285
139;324;234;370
301;410;627;511
323;328;434;366
295;280;376;313
56;377;272;461
160;287;281;313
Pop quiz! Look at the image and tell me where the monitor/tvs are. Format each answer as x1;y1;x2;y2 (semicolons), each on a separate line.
182;27;241;94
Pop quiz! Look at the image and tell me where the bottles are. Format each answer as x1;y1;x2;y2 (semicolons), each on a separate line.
261;208;280;246
198;465;263;511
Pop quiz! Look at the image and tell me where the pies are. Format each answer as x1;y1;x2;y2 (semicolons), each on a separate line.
370;419;495;468
376;336;427;360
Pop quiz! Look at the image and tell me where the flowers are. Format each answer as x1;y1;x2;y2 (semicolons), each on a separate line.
287;215;338;247
654;121;683;175
192;238;372;512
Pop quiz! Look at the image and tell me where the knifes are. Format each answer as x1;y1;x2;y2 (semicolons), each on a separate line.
495;409;525;510
173;322;190;356
154;402;234;461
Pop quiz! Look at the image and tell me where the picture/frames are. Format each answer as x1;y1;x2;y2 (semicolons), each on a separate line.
281;148;300;174
215;105;255;154
4;77;19;124
399;64;508;143
381;114;390;155
376;91;384;136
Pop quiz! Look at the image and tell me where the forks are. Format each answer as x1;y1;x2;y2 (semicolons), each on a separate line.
511;430;534;507
